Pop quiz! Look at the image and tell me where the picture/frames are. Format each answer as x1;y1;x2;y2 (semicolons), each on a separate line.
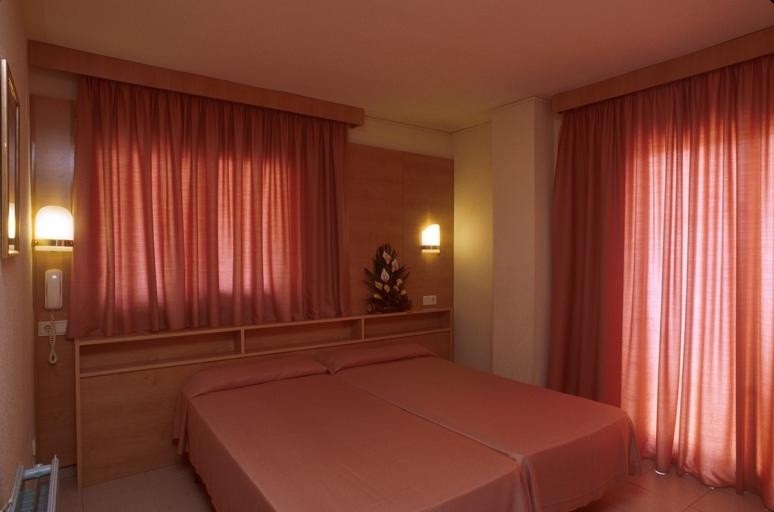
2;60;20;261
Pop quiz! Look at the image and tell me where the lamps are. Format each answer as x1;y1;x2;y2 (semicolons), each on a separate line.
421;223;441;254
35;206;74;253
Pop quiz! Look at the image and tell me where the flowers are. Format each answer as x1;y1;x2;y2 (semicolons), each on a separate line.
363;240;413;313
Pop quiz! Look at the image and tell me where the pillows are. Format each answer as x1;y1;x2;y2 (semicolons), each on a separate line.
184;336;441;400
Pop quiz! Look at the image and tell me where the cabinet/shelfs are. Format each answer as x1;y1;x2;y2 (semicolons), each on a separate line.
76;306;455;494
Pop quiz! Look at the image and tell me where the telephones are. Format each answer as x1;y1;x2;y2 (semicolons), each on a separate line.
44;269;64;311
